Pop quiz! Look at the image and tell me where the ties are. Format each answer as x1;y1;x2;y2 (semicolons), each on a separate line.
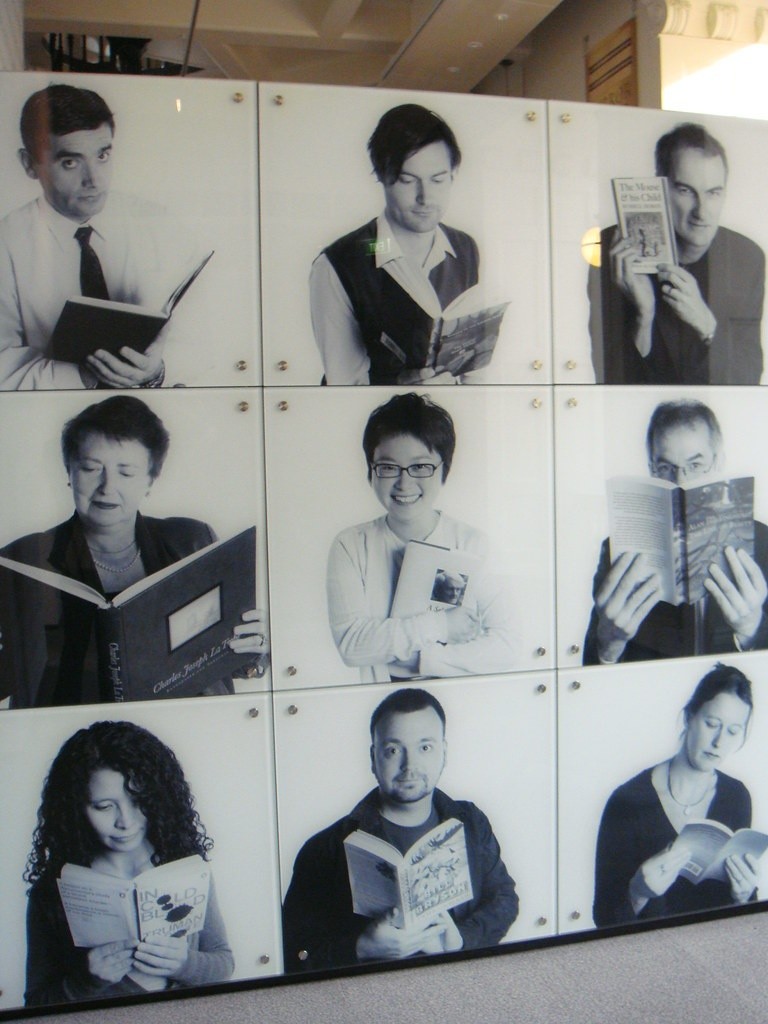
74;226;110;300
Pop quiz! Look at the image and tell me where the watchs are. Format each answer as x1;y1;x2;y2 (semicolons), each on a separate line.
139;361;165;388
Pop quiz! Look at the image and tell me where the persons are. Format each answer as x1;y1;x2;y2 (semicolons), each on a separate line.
22;722;235;1009
327;392;515;686
582;399;768;667
433;569;465;604
0;395;271;707
283;688;519;972
309;102;489;387
0;78;208;393
587;123;765;384
593;662;759;928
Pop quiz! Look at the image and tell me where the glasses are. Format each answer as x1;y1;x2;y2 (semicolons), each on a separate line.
371;457;444;478
648;453;716;478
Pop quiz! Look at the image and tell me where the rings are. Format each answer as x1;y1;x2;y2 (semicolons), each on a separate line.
668;287;673;295
259;636;265;646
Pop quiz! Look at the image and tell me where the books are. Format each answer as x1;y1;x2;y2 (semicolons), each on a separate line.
0;525;257;709
606;475;755;606
669;819;768;885
388;540;477;666
43;250;214;362
611;177;678;274
56;854;209;947
343;817;473;928
368;258;511;377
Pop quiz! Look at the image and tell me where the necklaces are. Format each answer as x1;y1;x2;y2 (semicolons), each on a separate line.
668;755;710;815
94;545;141;573
89;538;137;552
